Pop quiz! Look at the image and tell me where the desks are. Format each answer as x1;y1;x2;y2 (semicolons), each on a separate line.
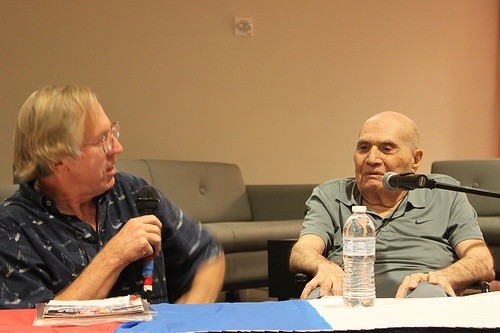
0;292;500;333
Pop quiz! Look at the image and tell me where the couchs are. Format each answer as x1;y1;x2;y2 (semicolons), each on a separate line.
106;159;500;299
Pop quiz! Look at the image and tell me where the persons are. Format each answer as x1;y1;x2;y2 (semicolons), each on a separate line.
0;83;225;312
289;111;495;300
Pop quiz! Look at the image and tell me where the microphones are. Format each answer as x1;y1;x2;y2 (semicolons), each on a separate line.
382;172;428;190
134;186;161;300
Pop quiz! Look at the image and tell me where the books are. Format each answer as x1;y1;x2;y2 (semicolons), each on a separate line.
33;295;158;327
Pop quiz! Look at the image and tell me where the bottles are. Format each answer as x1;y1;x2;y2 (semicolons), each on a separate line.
343;206;376;307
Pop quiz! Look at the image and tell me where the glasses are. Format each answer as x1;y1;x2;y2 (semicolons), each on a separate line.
83;120;120;154
